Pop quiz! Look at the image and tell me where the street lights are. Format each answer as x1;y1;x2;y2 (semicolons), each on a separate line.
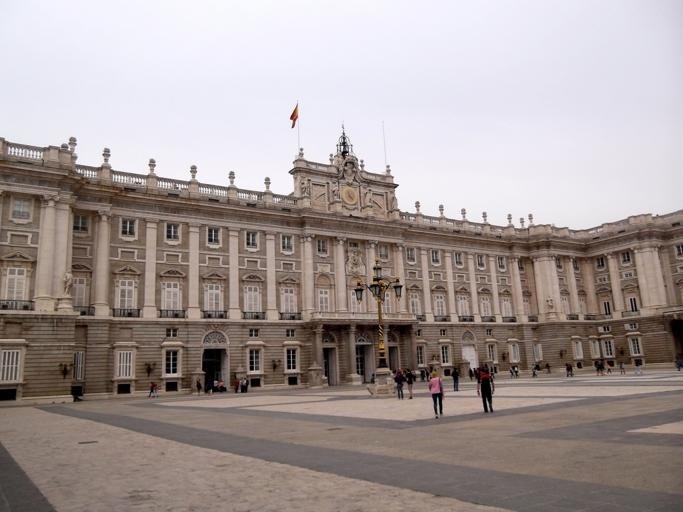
353;257;403;399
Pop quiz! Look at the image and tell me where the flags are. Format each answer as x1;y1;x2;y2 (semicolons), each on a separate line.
290;104;299;129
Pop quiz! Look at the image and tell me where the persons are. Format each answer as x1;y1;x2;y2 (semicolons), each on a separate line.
196;378;202;396
62;268;73;296
675;352;683;372
468;366;494;382
152;384;159;397
147;381;155;397
476;368;494;413
404;368;413;399
451;367;459;391
427;371;444;419
392;364;437;382
212;376;249;393
508;360;642;380
393;371;405;400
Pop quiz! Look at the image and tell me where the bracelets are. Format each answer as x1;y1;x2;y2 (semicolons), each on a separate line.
477;390;479;392
